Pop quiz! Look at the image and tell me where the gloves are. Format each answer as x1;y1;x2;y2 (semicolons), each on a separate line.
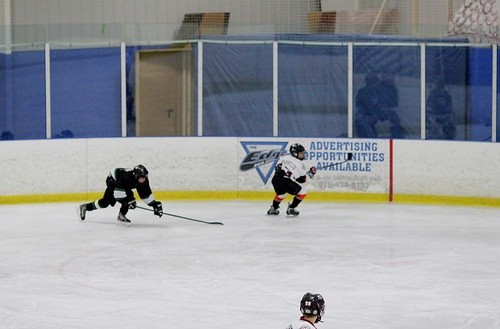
126;198;136;209
153;202;162;217
307;166;316;178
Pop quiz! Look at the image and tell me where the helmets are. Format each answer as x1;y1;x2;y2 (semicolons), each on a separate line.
290;144;305;160
300;293;325;323
133;165;148;182
382;69;394;80
432;76;445;89
366;74;379;81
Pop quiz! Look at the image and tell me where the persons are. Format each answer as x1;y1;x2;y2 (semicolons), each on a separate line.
357;70;407;137
79;165;164;223
285;292;326;329
124;82;136;122
55;129;74;138
425;76;459;140
266;142;317;217
0;131;14;140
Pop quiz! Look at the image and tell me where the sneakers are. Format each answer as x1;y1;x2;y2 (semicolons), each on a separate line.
287;203;299;216
117;209;131;225
267;206;279;216
77;204;89;220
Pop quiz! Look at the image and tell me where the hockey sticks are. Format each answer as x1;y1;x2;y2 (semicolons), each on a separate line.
317;153;353;169
136;206;223;224
